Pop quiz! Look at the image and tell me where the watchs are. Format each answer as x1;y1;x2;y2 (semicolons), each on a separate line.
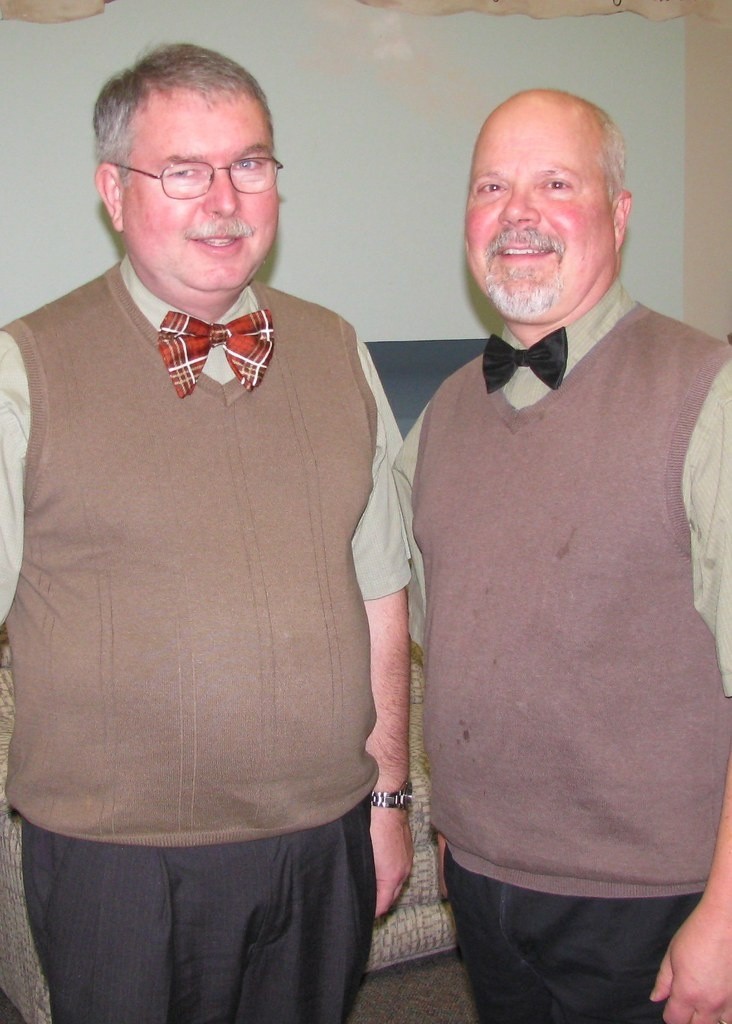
371;780;413;811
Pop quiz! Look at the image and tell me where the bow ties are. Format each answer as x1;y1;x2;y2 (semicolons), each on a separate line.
158;309;274;398
483;326;568;395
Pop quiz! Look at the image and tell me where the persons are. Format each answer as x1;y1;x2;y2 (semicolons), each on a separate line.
0;42;414;1024
393;83;732;1024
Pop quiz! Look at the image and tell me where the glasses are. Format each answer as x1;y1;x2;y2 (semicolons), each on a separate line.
108;156;283;200
